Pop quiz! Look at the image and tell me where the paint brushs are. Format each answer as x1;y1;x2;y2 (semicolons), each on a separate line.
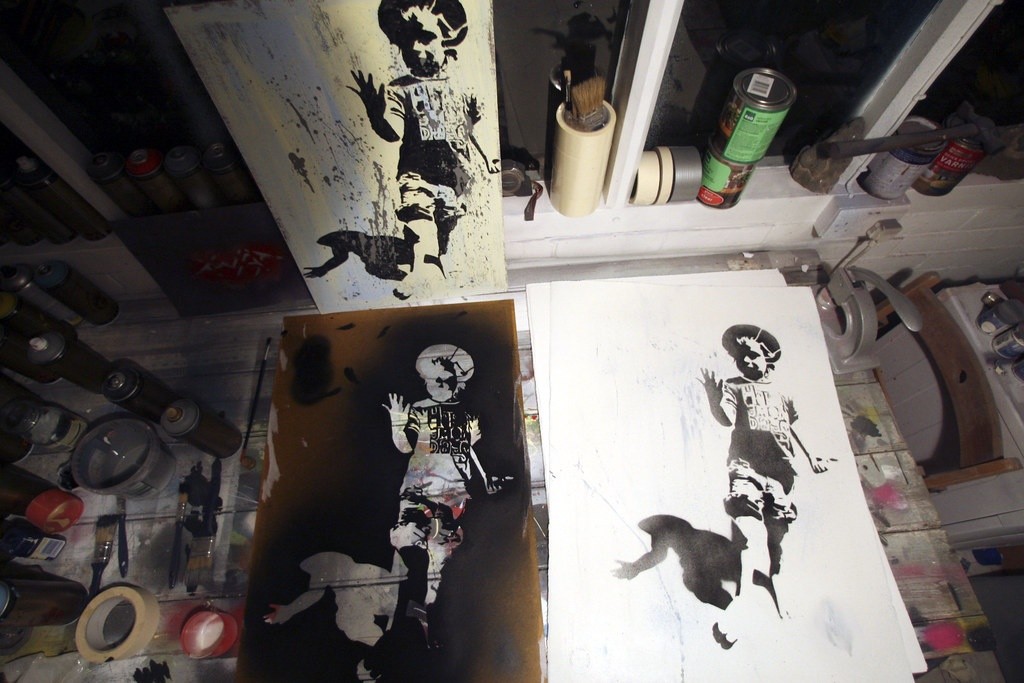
239;338;272;464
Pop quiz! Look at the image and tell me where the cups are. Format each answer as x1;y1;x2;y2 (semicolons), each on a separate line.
550;99;617;205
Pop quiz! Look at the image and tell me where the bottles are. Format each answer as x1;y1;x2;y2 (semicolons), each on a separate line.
126;149;194;213
0;518;67;561
203;143;266;204
86;152;154;218
976;298;1024;382
163;146;226;210
0;463;83;533
0;265;85;326
0;580;89;627
0;293;33;337
160;398;242;459
0;156;112;246
3;400;90;453
103;369;176;424
27;332;113;394
34;260;122;328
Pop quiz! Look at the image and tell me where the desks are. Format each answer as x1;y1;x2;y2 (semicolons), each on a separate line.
0;247;1005;683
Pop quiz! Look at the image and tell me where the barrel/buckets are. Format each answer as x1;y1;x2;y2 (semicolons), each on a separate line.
71;418;176;501
696;133;758;208
857;116;948;201
712;67;797;163
912;111;989;196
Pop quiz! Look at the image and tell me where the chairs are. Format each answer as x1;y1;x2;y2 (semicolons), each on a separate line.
873;270;1024;489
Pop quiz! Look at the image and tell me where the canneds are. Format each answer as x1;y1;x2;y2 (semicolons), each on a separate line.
711;68;796;164
858;115;947;200
912;134;984;197
696;132;757;209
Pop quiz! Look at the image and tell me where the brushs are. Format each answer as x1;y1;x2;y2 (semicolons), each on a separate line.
89;514;119;597
116;494;129;578
573;75;606;132
182;458;223;588
169;482;189;587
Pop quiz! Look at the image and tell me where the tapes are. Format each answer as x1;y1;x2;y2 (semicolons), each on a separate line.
75;584;162;666
628;144;703;207
549;100;617;219
